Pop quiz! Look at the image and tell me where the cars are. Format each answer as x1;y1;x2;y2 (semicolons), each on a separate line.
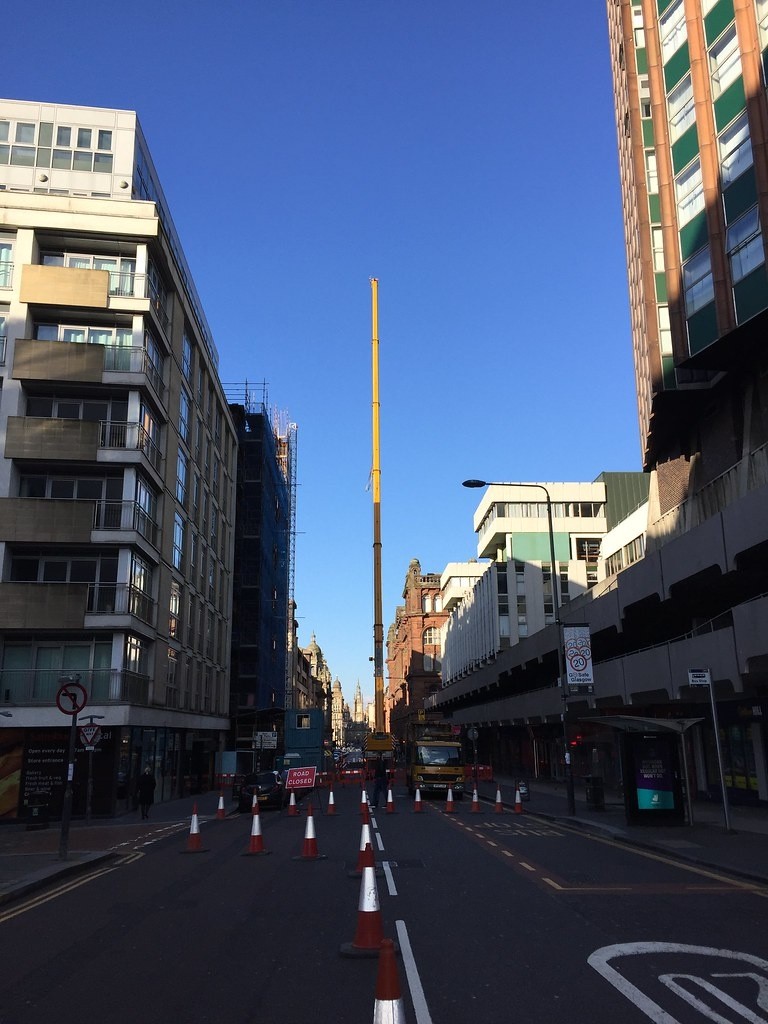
279;770;308;803
333;748;364;769
237;770;286;814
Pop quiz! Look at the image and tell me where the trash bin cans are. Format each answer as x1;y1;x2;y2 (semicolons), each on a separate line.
232;775;246;799
583;776;606;812
25;791;51;830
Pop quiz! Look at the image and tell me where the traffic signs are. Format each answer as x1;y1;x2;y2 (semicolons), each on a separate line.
687;668;711;687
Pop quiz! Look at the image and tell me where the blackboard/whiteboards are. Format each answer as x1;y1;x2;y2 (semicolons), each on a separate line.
514;779;529;801
18;742;92;818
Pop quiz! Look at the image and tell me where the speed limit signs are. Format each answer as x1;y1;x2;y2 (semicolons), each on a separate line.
565;637;592;672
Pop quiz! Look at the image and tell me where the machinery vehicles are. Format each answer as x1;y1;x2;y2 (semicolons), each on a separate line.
403;719;468;802
360;273;401;781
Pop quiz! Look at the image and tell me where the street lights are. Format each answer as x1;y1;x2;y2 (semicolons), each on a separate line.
76;713;106;823
460;479;577;816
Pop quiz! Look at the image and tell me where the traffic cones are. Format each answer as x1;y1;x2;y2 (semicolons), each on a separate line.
285;785;302;817
513;784;529;814
383;783;399;815
249;787;261;816
409;784;428;814
338;841;384;957
347;808;385;879
442;780;459;814
465;781;485;813
242;804;272;856
322;782;344;816
371;938;409;1024
177;799;210;853
291;802;330;860
214;788;234;822
491;784;509;816
358;783;374;815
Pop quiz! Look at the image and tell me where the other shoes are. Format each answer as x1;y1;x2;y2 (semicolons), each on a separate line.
382;804;387;809
368;804;378;808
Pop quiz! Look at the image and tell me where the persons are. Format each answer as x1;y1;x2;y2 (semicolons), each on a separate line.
371;755;389;809
138;767;156;820
